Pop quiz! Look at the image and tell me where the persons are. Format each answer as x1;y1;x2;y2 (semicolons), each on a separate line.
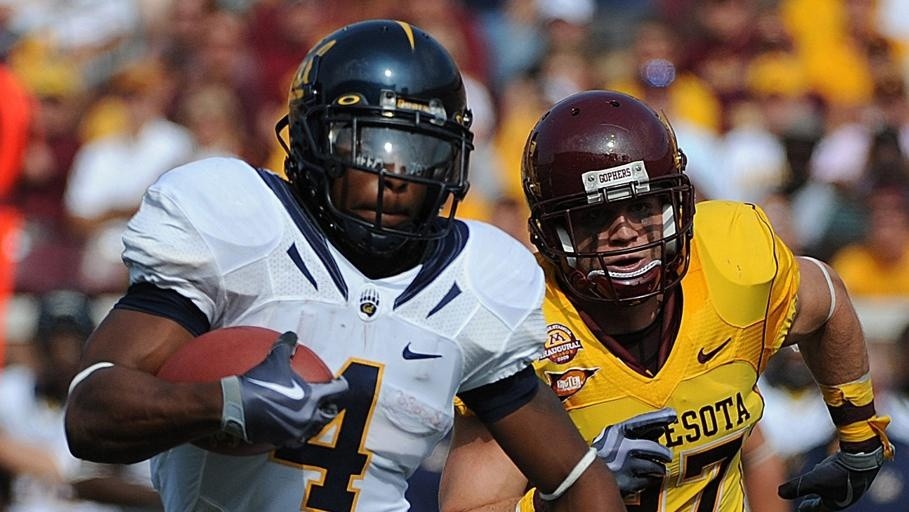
608;0;909;512
64;17;625;512
3;0;325;511
304;0;638;255
439;91;896;512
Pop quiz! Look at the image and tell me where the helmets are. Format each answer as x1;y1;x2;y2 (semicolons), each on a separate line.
522;88;695;306
274;20;473;269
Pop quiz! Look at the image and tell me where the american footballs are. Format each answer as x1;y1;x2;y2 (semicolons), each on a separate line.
158;327;331;455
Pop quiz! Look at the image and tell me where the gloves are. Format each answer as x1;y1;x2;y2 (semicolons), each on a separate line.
217;329;348;448
586;409;677;496
778;447;886;512
